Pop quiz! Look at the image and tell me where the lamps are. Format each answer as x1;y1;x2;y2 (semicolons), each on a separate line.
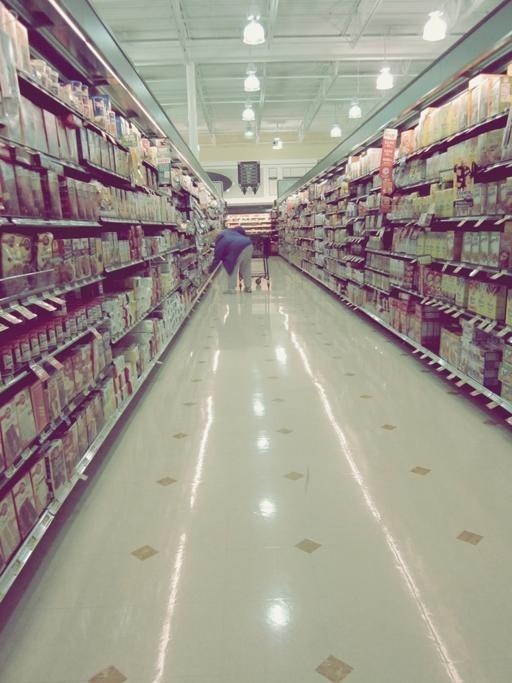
241;108;255;120
244;74;262;92
243;126;254;138
243;22;265;45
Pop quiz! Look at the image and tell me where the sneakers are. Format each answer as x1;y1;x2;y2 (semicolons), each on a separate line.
222;288;252;295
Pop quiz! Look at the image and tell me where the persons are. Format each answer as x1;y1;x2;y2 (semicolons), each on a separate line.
201;225;254;294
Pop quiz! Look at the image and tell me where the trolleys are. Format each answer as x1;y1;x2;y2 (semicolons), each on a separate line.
239;236;269;287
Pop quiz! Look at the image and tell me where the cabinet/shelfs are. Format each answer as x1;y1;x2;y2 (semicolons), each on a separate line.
279;28;512;416
0;2;227;605
225;211;279;255
